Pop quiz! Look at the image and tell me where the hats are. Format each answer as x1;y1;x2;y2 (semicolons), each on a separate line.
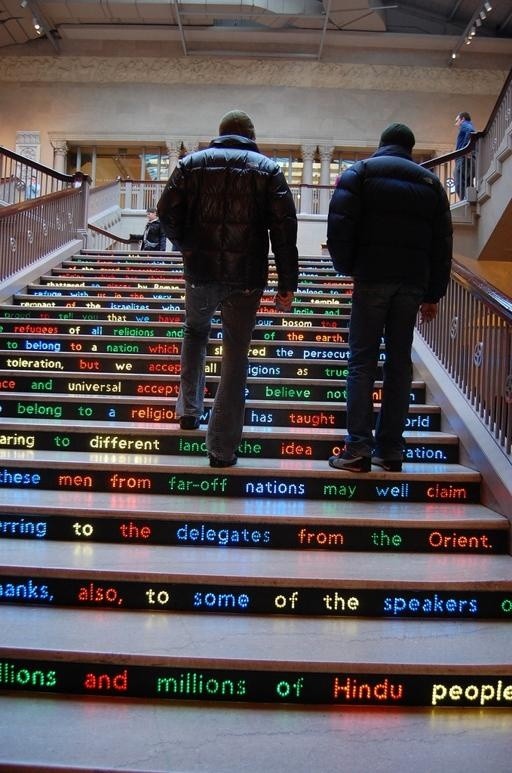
220;111;254;138
380;123;415;152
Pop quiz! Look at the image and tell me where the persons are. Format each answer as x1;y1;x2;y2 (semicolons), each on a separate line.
454;112;478;200
140;208;167;250
156;110;299;466
326;122;453;474
25;176;41;200
336;165;347;185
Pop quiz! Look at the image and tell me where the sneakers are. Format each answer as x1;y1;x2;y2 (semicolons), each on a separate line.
329;453;370;473
180;417;200;429
371;455;402;472
211;449;238;467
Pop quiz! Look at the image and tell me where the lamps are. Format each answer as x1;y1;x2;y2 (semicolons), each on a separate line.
449;0;492;60
19;0;61;54
